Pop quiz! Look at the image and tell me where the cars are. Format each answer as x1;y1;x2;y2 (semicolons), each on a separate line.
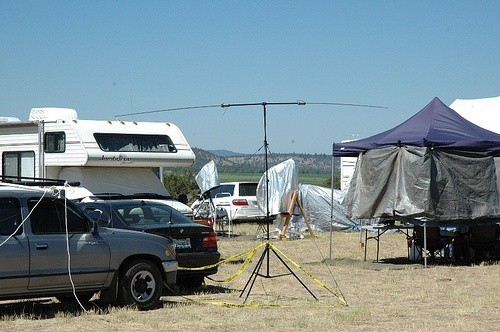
73;192;220;291
0;177;178;310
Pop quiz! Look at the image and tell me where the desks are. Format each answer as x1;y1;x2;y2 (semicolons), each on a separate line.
363;225;418;262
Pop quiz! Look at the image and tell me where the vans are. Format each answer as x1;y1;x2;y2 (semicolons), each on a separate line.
191;182;274;226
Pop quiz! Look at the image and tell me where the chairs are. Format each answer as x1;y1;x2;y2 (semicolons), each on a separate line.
213;205;233;238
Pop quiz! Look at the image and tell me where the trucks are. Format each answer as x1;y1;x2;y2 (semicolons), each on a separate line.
0;107;194;200
